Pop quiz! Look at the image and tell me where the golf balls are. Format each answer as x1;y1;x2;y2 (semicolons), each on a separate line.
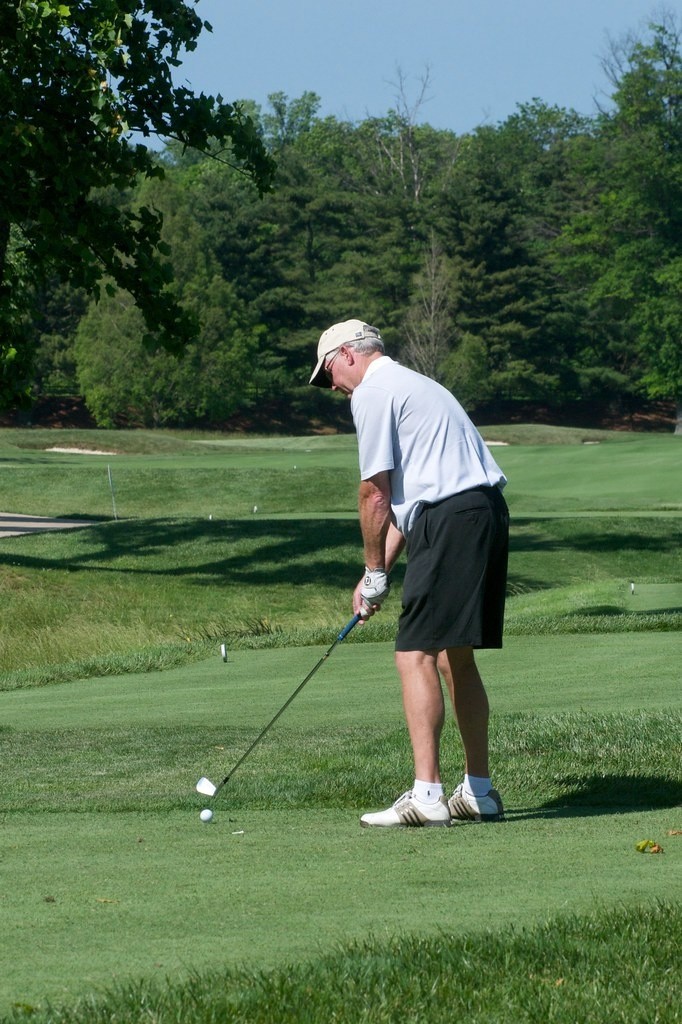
200;809;213;823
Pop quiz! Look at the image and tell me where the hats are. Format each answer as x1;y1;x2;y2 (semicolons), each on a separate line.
308;319;382;388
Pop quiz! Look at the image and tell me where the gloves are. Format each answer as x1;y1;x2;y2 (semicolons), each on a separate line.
360;566;391;608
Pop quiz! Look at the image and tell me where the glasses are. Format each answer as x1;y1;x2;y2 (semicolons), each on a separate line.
324;351;341;379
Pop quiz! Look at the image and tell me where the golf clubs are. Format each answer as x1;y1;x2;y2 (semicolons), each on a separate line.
195;605;369;799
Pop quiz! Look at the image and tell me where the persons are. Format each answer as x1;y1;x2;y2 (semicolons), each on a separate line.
308;318;510;827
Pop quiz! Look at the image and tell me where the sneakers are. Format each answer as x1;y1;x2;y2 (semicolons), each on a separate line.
448;789;504;821
360;795;453;828
